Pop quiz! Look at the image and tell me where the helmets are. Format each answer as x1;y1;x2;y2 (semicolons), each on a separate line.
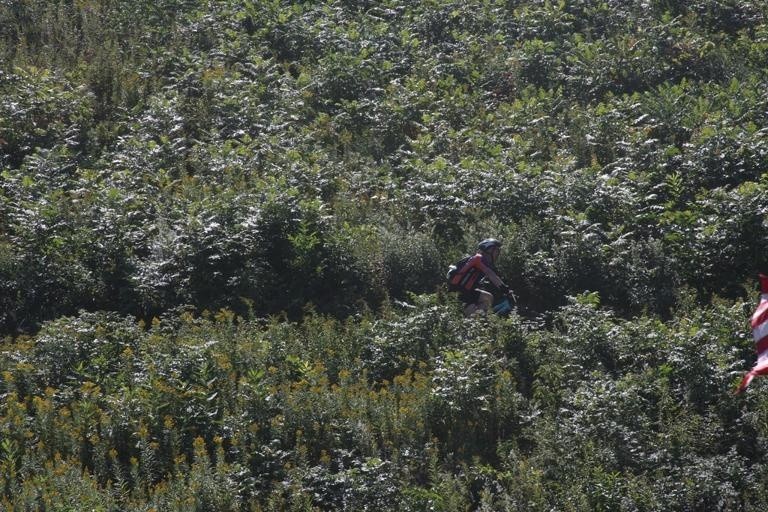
478;238;503;251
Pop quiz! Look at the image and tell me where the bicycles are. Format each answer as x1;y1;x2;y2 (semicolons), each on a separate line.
457;285;520;321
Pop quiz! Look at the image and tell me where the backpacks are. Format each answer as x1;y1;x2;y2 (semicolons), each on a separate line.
446;256;470;281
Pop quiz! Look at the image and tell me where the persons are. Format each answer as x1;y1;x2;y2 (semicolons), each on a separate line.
446;238;514;317
